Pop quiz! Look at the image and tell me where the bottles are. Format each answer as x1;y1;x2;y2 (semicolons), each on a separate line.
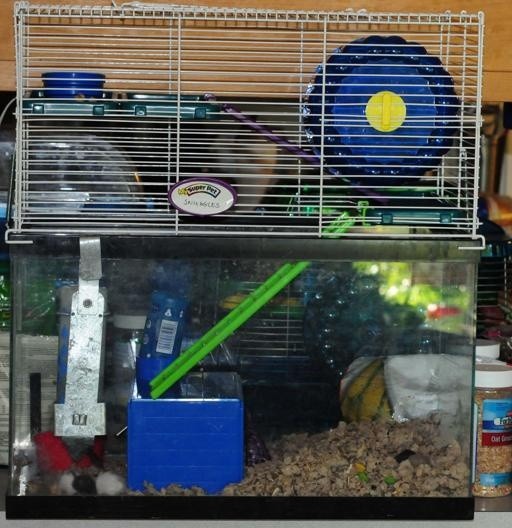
135;259;196;398
471;338;511;498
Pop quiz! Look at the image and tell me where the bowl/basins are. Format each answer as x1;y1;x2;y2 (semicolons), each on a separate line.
385;354;506;420
